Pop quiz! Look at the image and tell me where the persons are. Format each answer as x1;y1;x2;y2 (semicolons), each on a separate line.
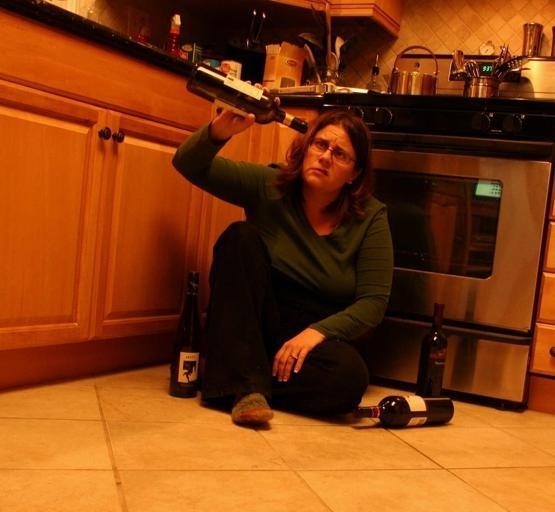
171;81;396;424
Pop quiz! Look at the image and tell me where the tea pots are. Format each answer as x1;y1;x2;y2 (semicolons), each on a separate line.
386;45;438;97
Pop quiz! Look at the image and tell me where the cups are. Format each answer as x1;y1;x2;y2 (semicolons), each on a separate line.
463;77;501;99
521;21;544;58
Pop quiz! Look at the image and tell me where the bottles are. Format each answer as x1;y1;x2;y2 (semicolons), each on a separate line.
414;301;464;392
185;60;309;135
366;52;381;92
353;393;455;426
167;269;203;398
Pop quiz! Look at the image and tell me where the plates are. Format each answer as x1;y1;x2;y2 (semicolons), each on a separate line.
480;41;495;56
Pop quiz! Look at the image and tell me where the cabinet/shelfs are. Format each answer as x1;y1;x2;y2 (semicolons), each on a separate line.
528;165;555;381
0;75;210;355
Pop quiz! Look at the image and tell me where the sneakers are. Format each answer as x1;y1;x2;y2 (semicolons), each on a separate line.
231;392;274;424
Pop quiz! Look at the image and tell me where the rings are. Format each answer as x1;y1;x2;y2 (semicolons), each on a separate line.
288;353;297;359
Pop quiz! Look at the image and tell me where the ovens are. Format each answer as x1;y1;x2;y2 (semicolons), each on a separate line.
329;97;551;412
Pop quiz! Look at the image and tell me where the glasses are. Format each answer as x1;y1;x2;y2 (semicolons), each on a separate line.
309;137;357;165
